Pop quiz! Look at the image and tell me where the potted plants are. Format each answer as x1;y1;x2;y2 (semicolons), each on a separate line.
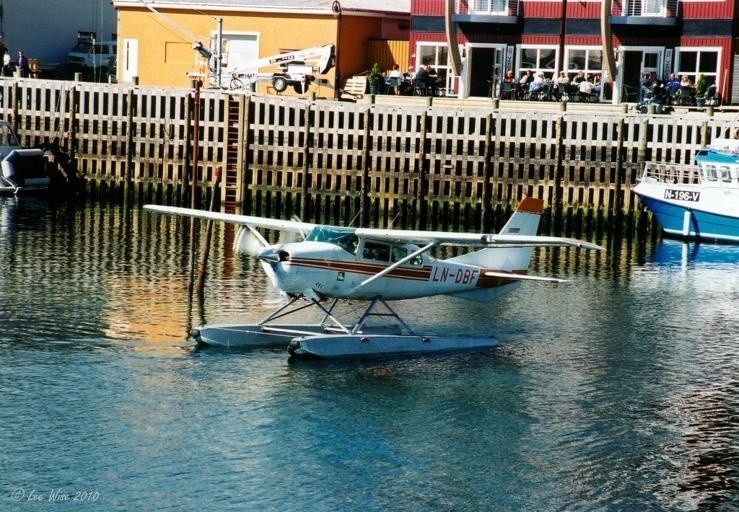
695;73;704;107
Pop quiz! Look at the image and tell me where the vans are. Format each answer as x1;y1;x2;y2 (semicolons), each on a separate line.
67;39;117;68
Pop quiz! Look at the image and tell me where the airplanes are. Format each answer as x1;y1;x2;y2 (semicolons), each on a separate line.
143;195;606;361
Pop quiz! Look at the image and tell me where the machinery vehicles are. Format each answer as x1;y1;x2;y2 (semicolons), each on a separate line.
185;30;335;94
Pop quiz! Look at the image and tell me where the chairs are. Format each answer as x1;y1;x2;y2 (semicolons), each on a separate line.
386;75;428;95
500;80;600;103
622;84;638;103
705;87;720;106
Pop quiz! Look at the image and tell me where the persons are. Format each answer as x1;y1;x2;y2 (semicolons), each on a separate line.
501;70;599;100
367;63;438;95
640;71;697;105
0;34;29;78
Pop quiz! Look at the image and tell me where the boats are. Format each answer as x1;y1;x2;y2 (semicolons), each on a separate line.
633;138;739;244
0;119;58;194
